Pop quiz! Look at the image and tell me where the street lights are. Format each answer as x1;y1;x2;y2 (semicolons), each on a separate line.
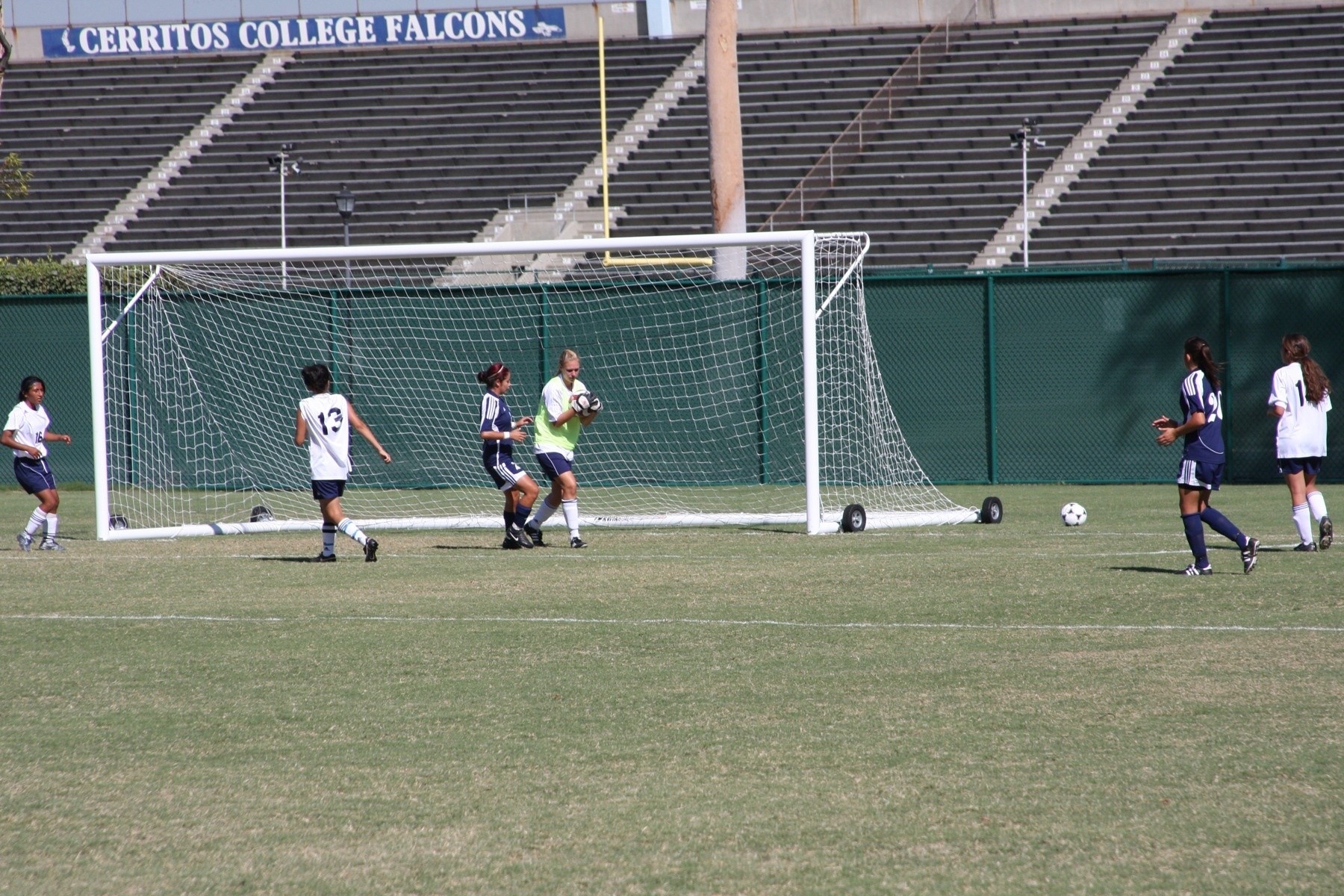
1009;117;1047;273
267;142;304;290
333;184;356;463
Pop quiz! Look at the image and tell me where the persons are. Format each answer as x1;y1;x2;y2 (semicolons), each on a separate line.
293;364;392;562
1152;336;1261;577
523;349;604;548
0;376;72;552
1267;333;1334;551
478;363;533;549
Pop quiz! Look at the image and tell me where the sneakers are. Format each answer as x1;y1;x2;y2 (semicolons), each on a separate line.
16;533;36;552
502;536;526;550
571;537;588;549
310;551;336;562
1176;563;1213;575
506;523;533;549
39;539;65;550
363;537;378;562
524;522;546;547
1294;542;1317;552
1319;516;1333;550
1241;537;1260;574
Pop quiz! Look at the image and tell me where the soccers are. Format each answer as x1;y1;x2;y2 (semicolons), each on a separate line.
1061;502;1087;527
569;389;591;418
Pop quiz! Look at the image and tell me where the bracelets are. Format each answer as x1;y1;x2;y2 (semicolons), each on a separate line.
511;421;516;428
504;431;510;439
